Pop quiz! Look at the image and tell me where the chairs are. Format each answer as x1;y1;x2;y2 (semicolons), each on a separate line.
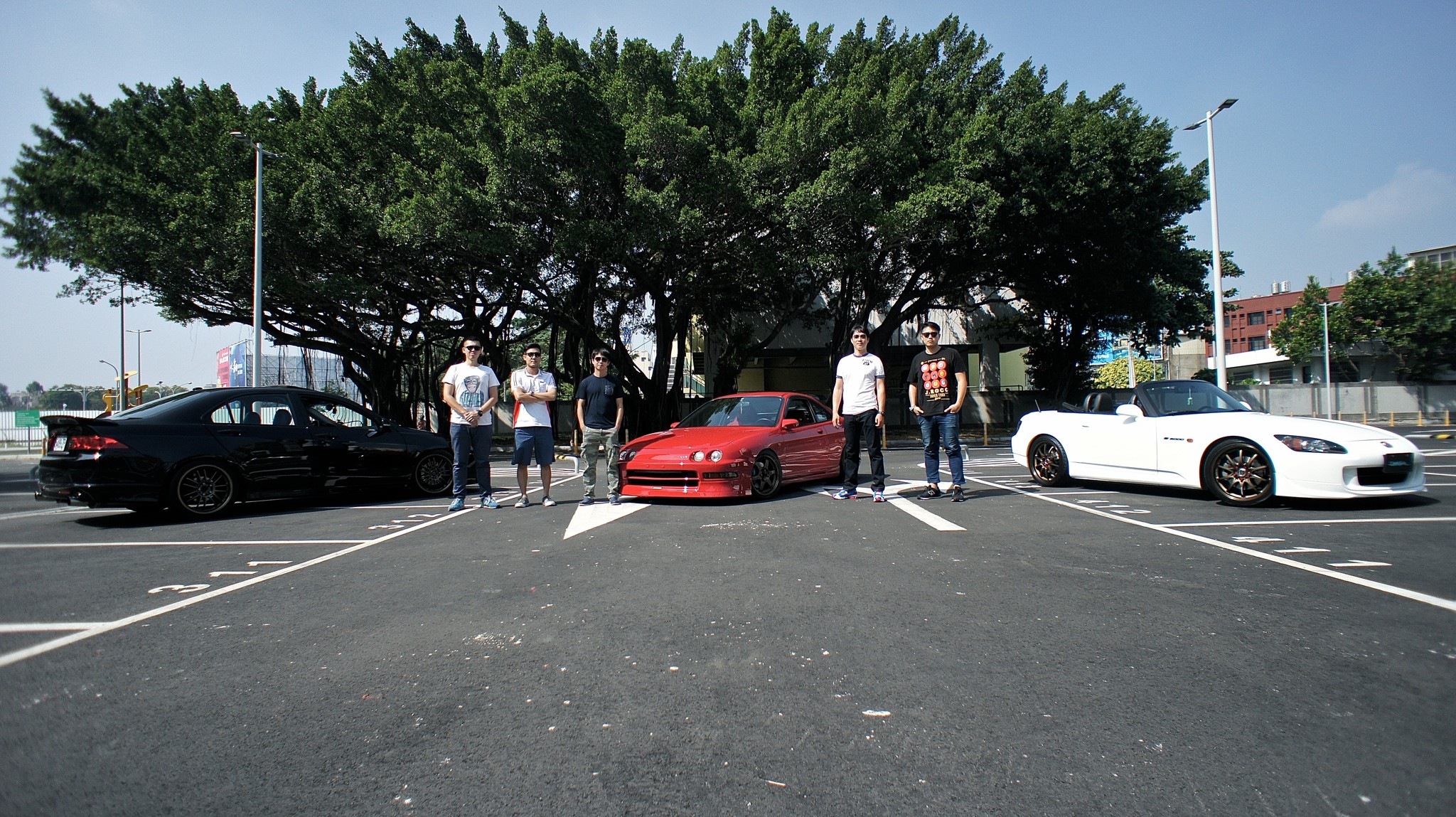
273;409;293;425
1091;392;1116;414
243;412;261;424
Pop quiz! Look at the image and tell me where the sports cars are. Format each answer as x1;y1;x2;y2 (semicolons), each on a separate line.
1010;379;1428;510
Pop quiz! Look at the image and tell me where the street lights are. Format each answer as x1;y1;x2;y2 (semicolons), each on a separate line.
100;277;153;413
1181;97;1241;409
1312;301;1342;420
229;130;280;412
153;380;192;400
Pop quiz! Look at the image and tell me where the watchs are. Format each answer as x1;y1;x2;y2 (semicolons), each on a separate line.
878;411;887;416
476;410;483;417
909;405;917;412
529;391;534;396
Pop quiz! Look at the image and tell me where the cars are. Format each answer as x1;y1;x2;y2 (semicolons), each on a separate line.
616;392;846;497
34;383;477;521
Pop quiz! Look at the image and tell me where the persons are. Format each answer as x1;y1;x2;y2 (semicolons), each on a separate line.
831;323;886;503
575;347;626;506
1004;388;1010;391
510;342;557;508
440;336;502;512
906;321;969;502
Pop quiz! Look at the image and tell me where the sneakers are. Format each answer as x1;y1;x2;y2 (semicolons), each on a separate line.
917;485;942;500
448;498;465;511
578;497;594;505
480;495;500;509
609;496;621;505
831;488;857;499
951;487;964;501
873;491;884;502
542;495;555;506
514;497;529;508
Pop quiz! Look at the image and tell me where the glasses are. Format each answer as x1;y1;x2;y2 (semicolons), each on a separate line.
594;357;609;362
852;335;866;339
464;345;482;351
922;332;939;337
525;352;541;358
